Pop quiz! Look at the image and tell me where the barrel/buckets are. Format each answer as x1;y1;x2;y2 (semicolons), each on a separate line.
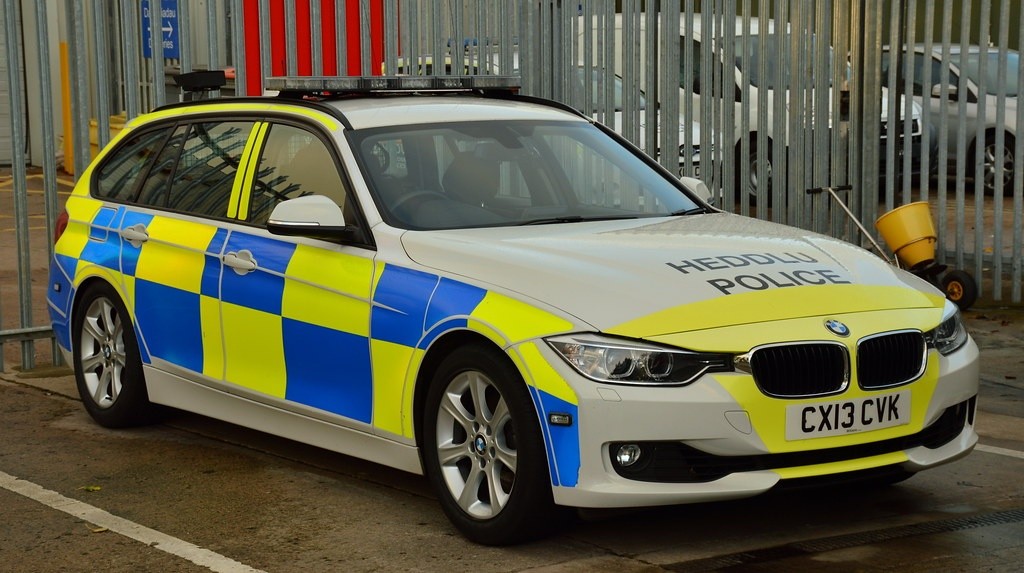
875;201;937;269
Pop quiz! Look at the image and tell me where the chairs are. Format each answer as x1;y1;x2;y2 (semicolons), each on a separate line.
414;151;505;230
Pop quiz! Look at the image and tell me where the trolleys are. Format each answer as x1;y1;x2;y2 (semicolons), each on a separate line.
802;184;975;313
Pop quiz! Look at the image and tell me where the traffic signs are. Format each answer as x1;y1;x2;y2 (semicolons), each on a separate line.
140;0;179;59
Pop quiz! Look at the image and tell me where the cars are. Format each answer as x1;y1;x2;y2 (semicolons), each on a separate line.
571;11;938;209
847;43;1019;200
380;37;730;209
45;64;979;551
